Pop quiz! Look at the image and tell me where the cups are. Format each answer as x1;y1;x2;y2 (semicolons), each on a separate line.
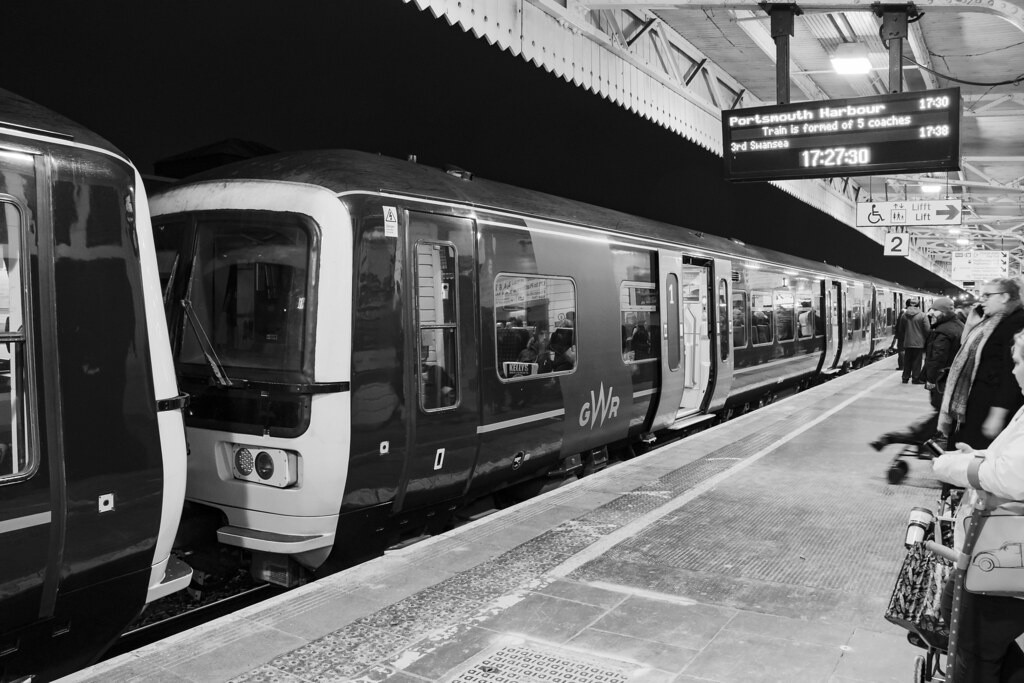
905;507;934;547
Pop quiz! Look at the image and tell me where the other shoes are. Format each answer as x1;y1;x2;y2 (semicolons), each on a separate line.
912;379;924;384
895;367;903;370
902;379;908;383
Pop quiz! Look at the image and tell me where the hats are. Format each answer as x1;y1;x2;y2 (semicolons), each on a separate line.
910;297;920;305
930;297;954;313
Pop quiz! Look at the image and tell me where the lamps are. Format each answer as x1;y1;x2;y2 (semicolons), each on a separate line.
830;41;873;75
921;181;943;193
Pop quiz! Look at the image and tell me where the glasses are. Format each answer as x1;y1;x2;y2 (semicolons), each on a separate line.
981;292;1004;297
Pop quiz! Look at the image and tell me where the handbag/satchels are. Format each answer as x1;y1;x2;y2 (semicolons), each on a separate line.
963;512;1023;596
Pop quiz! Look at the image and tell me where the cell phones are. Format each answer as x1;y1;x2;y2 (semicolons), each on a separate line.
975;304;984;318
923;438;945;458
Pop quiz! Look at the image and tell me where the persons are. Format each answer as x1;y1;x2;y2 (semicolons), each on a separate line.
502;319;573;379
890;299;979;384
799;301;814;337
932;332;1024;683
936;278;1024;517
733;309;743;326
918;298;964;410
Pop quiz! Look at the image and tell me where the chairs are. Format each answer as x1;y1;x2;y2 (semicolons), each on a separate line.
734;326;745;345
757;325;771;343
742;325;760;344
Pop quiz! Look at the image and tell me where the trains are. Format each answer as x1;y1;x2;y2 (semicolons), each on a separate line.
0;85;949;683
